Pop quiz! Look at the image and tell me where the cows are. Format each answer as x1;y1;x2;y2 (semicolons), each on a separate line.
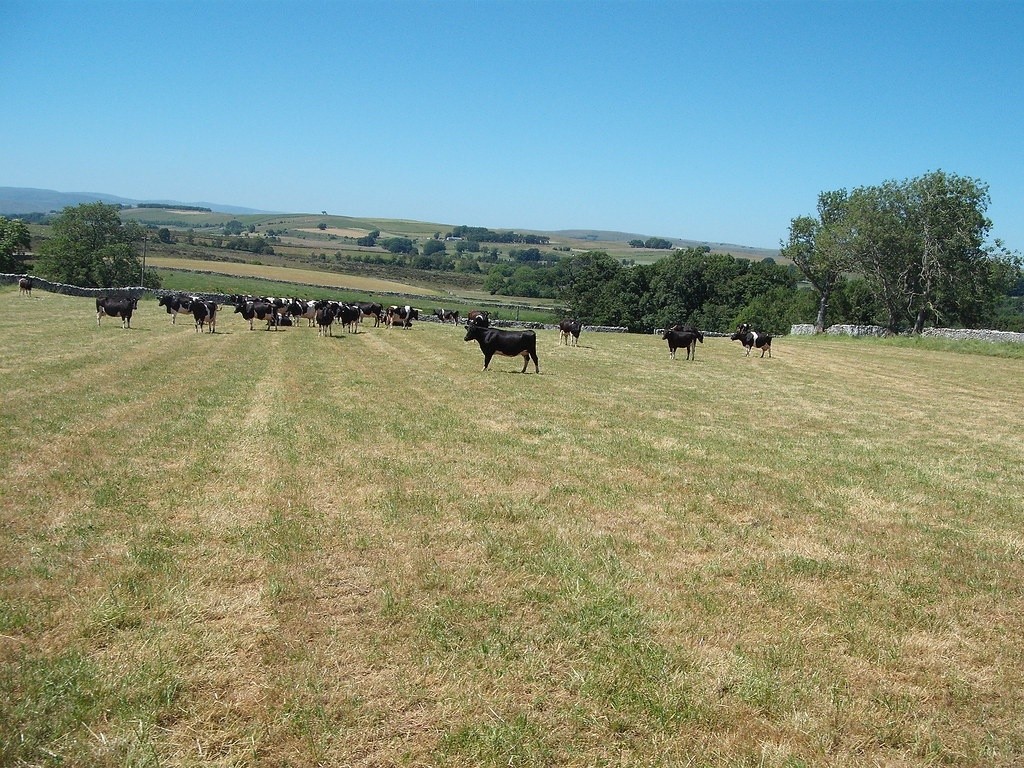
730;321;773;360
662;321;705;361
95;296;140;329
463;309;540;378
430;306;459;325
155;288;420;338
18;278;34;297
558;318;583;347
